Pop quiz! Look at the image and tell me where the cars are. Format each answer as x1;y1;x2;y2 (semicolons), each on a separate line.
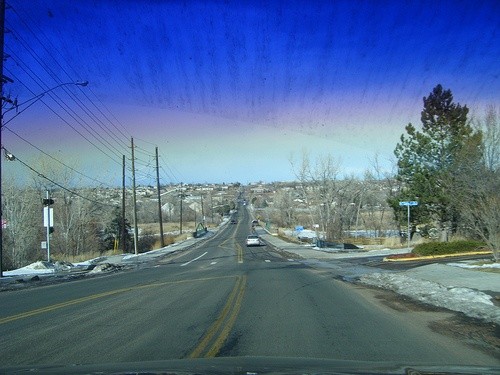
246;235;260;246
230;218;237;224
252;219;258;226
242;200;247;206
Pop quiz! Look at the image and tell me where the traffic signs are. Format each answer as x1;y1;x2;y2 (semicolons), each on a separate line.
398;200;418;206
